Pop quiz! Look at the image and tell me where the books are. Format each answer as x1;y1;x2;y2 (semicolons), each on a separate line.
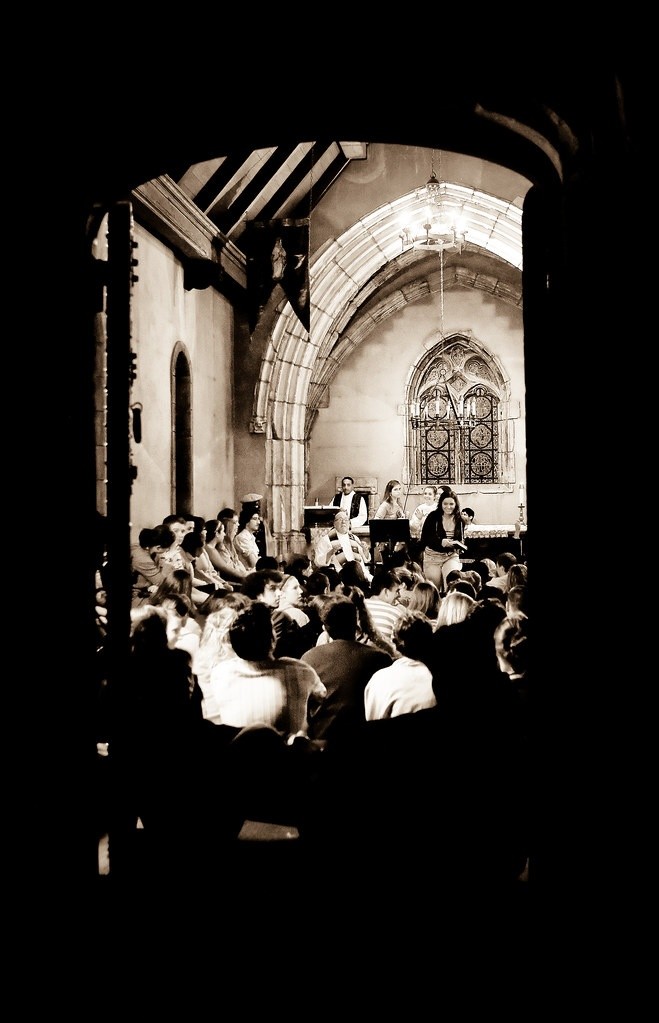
449;540;467;552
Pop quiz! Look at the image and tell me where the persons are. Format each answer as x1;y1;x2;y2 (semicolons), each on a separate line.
436;486;452;499
418;492;466;593
232;509;262;569
328;477;367;530
360;572;408;644
409;486;440;531
314;512;374;582
460;508;475;525
95;506;529;742
374;480;403;520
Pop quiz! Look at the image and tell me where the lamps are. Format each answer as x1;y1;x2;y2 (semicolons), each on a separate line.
517;483;525;504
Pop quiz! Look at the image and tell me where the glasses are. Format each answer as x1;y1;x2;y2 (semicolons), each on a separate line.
335;517;349;522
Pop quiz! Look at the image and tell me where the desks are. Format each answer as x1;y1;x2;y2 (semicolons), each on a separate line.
353;524;527;563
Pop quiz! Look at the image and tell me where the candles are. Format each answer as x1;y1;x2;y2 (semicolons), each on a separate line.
414;403;420;416
436;395;440;414
467;399;471;417
446;399;450;420
459;395;464;414
411;400;414;415
471;397;476;415
